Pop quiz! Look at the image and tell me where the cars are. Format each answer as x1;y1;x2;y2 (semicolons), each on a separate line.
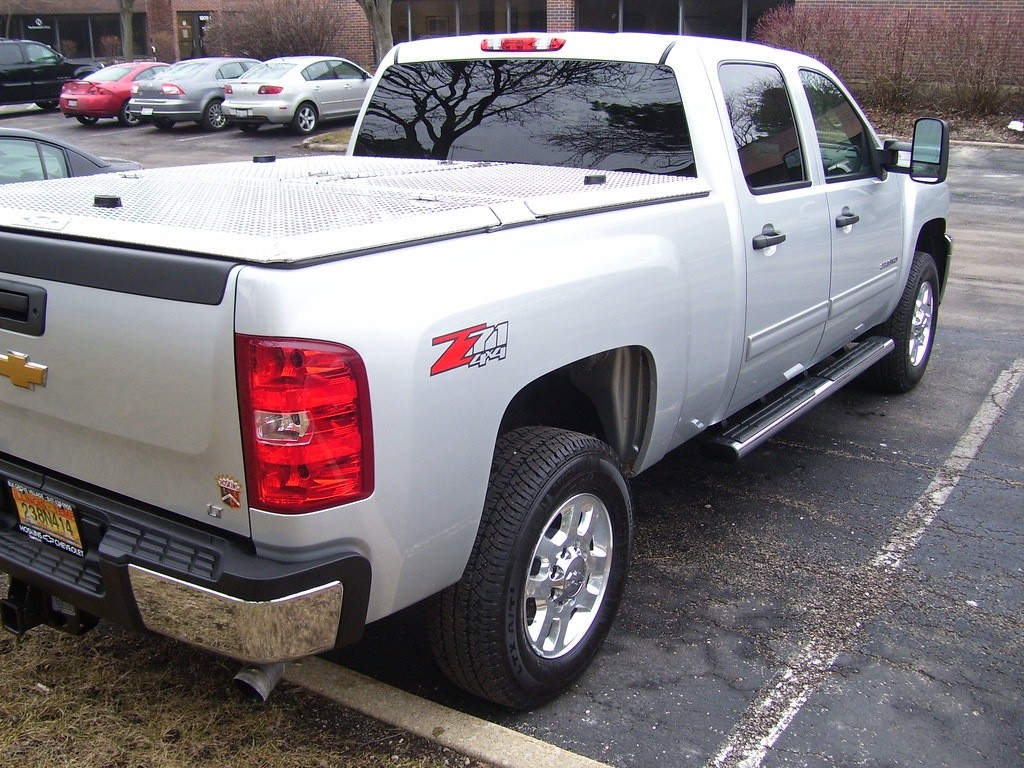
59;62;175;127
220;56;376;135
129;57;262;133
0;128;144;185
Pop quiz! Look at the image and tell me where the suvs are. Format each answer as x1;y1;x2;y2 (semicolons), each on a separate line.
0;37;105;109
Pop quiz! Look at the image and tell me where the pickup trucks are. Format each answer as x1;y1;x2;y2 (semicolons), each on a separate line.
0;31;953;711
